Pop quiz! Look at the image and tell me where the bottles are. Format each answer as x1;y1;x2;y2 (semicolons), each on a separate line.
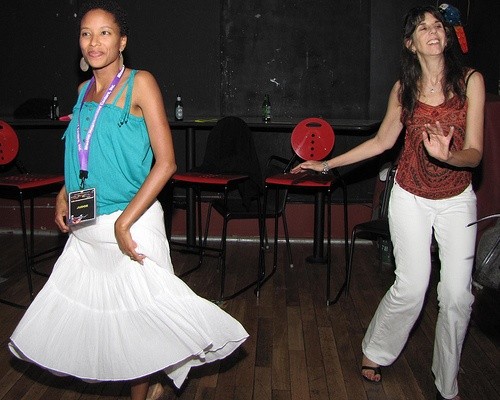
174;93;184;121
261;93;271;125
50;96;59;120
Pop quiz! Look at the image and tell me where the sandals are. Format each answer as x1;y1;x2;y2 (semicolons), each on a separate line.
437;391;459;400
362;366;381;385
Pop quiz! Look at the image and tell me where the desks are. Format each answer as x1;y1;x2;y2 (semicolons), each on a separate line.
0;115;385;265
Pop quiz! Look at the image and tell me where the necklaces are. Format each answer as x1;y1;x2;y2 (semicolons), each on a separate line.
431;89;434;93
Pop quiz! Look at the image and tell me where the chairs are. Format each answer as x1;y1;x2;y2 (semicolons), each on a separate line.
1;115;442;309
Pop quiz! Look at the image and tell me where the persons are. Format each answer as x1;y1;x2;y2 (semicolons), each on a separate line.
8;0;250;400
291;5;485;400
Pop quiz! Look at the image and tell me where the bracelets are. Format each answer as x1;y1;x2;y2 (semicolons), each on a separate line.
322;160;328;174
